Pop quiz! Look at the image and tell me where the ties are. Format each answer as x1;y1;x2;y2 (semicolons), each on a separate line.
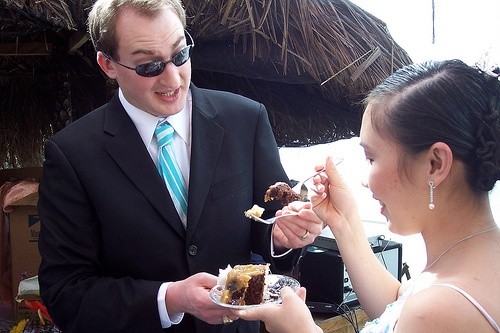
156;122;188;230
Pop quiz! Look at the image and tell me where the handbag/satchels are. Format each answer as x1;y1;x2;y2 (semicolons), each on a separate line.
15;275;40;303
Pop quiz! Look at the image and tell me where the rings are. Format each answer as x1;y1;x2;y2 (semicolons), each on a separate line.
223;316;233;325
301;230;310;239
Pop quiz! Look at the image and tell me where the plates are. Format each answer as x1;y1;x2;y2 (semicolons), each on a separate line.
208;274;300;310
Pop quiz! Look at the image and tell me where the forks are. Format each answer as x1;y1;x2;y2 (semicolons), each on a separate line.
290;158;342;196
244;212;299;225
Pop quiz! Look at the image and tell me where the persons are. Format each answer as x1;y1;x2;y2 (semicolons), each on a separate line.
229;59;499;333
39;0;323;333
289;179;311;204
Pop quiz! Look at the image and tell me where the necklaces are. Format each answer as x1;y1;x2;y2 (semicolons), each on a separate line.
421;225;498;273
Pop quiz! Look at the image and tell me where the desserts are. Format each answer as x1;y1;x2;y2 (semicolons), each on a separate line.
245;205;264;219
264;182;302;205
220;264;271;305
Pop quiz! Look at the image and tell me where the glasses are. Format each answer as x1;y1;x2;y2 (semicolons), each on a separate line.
101;28;196;78
